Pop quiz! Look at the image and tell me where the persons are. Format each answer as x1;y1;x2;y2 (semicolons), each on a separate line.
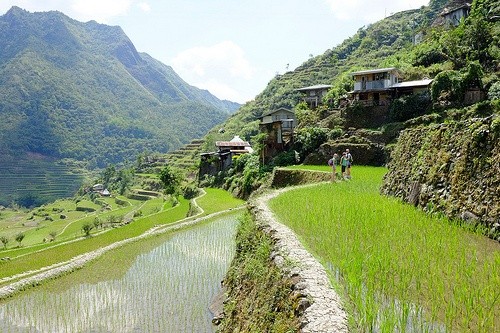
340;152;347;178
345;148;353;174
328;153;338;182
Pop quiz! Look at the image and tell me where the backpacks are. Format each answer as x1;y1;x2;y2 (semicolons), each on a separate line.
328;158;333;166
